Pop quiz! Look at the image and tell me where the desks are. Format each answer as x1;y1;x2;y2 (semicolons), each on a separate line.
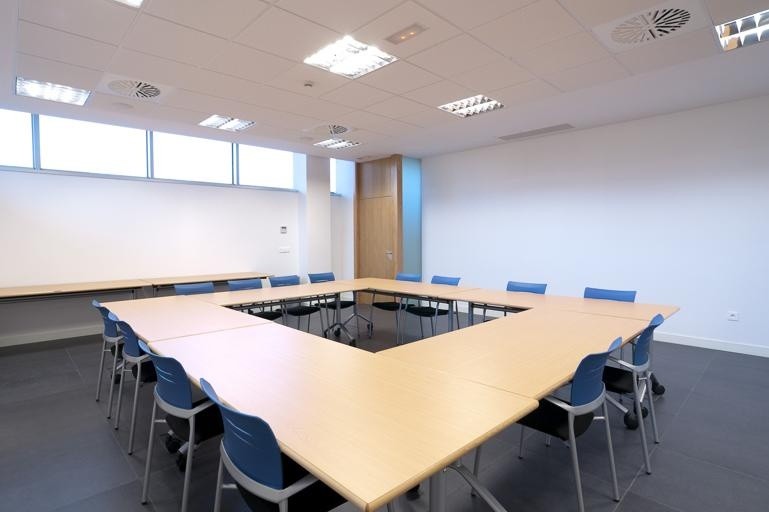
0;278;159;300
